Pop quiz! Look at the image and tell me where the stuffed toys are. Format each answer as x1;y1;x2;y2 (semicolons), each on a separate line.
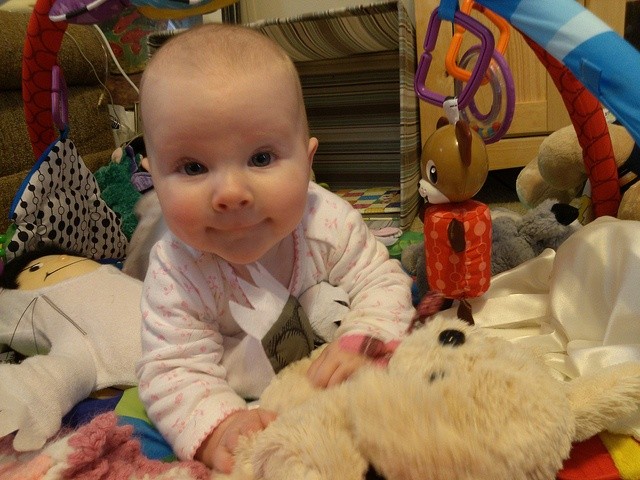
1;247;145;452
400;197;582;306
412;6;495;325
228;321;640;480
515;121;640;222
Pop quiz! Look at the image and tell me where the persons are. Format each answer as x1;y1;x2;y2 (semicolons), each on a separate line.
134;21;416;473
